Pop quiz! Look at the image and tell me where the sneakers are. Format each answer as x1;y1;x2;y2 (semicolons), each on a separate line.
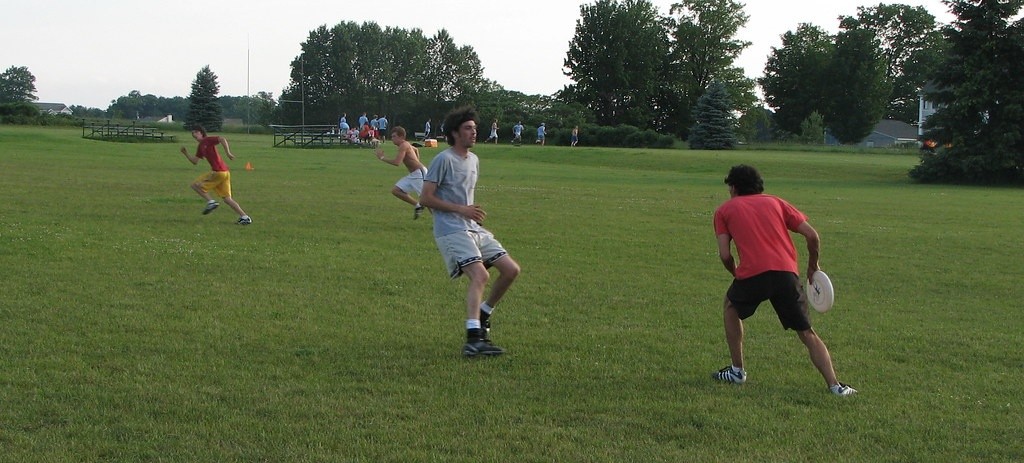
829;381;858;396
202;201;220;215
236;217;252;225
480;314;491;335
712;365;747;385
463;338;504;357
414;206;426;219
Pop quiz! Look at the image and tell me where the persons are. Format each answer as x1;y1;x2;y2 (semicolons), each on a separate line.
419;110;520;356
339;112;388;148
373;126;434;220
423;117;431;142
484;119;499;145
711;164;858;396
536;123;547;146
511;121;524;145
571;126;578;147
180;124;252;225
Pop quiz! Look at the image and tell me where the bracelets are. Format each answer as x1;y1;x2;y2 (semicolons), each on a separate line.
381;156;385;160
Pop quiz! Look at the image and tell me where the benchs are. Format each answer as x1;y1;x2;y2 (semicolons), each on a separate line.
415;132;445;140
79;119;176;142
268;123;367;148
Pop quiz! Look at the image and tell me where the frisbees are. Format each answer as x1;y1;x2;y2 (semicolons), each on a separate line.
805;269;834;312
